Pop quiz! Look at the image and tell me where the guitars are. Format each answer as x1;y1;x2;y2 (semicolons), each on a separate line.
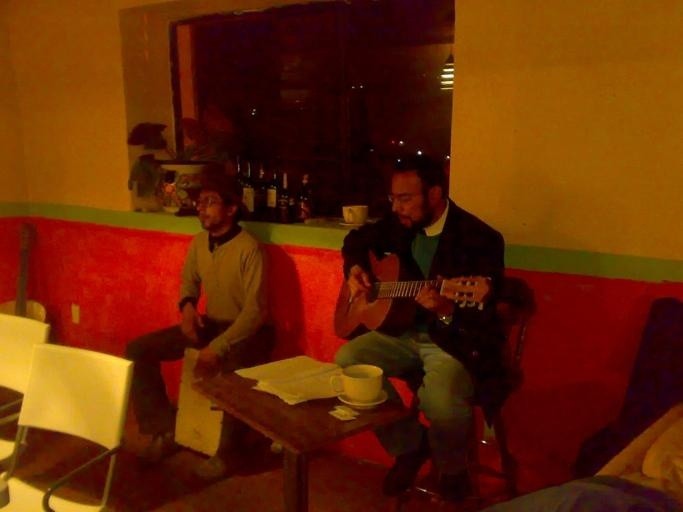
332;250;491;340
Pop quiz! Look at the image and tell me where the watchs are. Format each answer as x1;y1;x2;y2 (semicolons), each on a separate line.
438;313;454;325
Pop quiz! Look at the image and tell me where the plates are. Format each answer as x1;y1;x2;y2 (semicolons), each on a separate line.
335;390;388;410
338;222;364;227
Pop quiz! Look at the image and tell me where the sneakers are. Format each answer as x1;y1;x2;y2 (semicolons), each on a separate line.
384;429;431;495
439;470;470;502
136;432;181;464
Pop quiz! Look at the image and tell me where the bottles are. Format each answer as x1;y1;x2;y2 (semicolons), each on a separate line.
235;155;314;221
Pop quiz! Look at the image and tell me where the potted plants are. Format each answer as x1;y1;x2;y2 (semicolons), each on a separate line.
128;118;218;214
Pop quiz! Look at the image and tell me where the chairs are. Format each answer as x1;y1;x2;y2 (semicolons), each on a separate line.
1;344;136;512
0;312;51;469
396;276;535;509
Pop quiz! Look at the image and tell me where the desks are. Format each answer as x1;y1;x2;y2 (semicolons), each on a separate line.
192;362;414;512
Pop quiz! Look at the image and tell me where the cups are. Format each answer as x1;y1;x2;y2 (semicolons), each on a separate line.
342;205;369;224
329;364;385;402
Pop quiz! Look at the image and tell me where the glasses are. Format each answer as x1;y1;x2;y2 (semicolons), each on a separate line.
192;198;216;208
388;195;414;203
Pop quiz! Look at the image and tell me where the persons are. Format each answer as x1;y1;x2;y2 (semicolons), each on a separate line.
340;155;506;504
121;177;278;485
